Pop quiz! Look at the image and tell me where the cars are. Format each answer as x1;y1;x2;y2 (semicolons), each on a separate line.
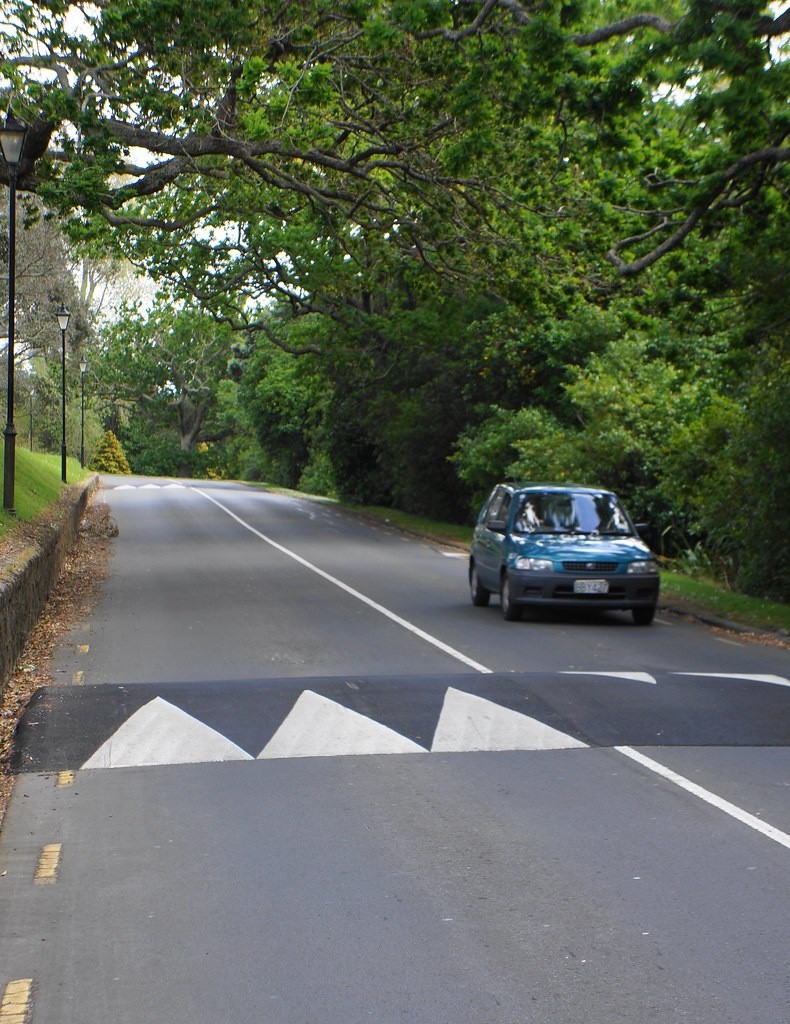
468;483;660;626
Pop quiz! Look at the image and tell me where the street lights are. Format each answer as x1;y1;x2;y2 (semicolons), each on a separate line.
55;301;71;484
0;104;28;517
78;354;88;469
